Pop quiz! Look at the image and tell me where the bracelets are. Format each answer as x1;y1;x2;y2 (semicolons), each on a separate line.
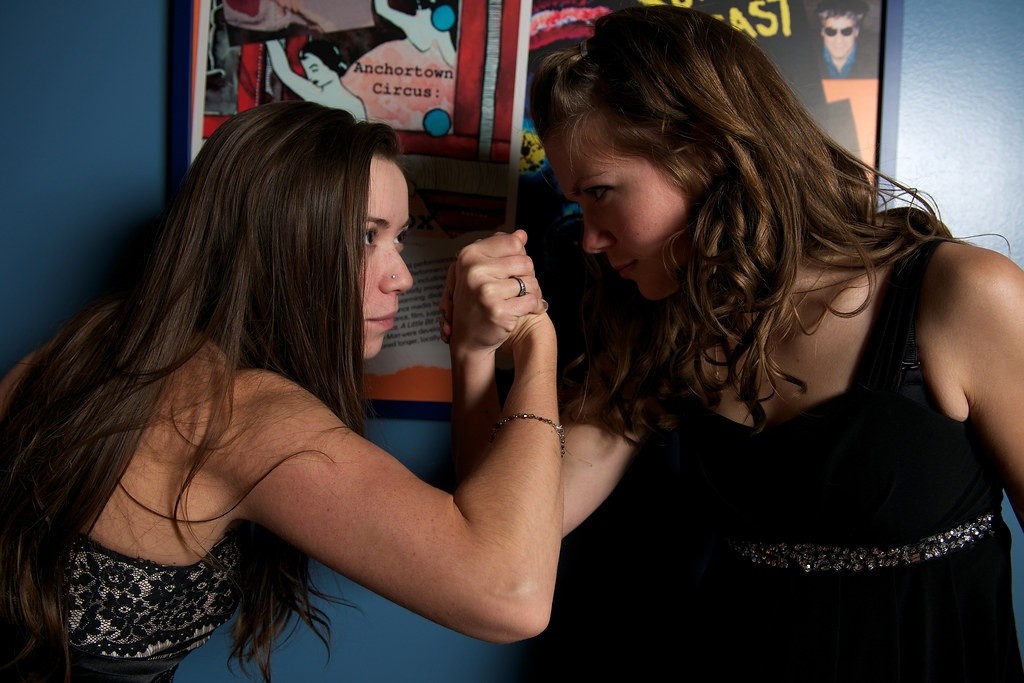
489;413;566;458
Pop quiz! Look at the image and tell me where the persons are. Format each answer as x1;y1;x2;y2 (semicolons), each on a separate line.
803;3;879;79
0;101;566;682
438;5;1022;682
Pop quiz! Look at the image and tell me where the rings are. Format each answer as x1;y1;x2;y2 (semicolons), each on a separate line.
510;276;527;296
